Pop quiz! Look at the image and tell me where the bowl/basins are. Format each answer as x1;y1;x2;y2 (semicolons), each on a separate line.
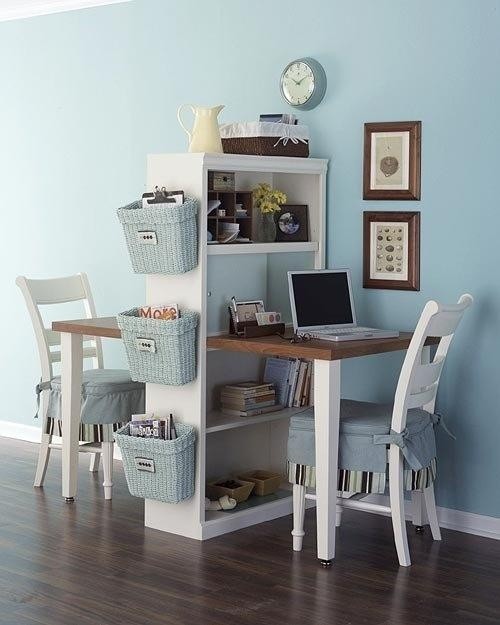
219;222;240;243
207;469;282;503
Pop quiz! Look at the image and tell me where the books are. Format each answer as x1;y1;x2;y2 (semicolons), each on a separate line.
220;354;314;417
295;118;302;124
259;114;289;124
127;410;178;442
287;113;296;125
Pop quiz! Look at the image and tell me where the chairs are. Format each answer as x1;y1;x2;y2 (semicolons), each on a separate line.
284;292;479;567
14;271;145;502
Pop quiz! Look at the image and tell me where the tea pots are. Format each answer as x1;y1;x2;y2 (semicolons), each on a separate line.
176;103;225;154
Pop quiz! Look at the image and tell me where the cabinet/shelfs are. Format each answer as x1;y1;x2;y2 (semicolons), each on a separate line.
137;151;335;545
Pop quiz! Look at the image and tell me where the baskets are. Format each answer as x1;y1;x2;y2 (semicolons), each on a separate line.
113;420;194;504
118;197;198;275
117;306;198;387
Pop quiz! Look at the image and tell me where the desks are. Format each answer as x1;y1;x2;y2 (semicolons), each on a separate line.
51;316;123;504
207;324;443;566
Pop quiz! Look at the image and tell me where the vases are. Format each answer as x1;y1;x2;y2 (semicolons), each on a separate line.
251;206;277;243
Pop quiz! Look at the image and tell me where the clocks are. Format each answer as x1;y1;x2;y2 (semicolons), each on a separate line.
279;57;328;111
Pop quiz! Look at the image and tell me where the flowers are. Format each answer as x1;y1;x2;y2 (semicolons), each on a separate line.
251;180;288;215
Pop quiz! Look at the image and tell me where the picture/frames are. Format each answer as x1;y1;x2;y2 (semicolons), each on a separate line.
358;118;424;293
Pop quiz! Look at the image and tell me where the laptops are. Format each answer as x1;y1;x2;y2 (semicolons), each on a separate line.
286;268;400;342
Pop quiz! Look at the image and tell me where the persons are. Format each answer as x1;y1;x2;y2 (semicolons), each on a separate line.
287;214;300;234
282;218;294;233
